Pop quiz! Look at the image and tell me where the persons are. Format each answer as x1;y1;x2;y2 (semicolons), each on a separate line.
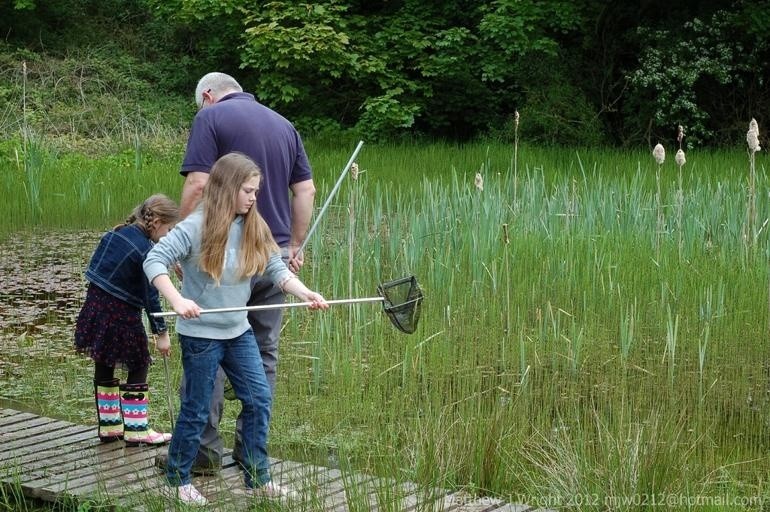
155;71;317;478
74;192;181;447
143;152;329;508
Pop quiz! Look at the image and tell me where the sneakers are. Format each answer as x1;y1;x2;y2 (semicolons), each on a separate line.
246;481;288;498
164;483;209;507
156;448;223;476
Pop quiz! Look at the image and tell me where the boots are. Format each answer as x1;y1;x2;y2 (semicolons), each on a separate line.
93;378;124;442
118;382;173;447
